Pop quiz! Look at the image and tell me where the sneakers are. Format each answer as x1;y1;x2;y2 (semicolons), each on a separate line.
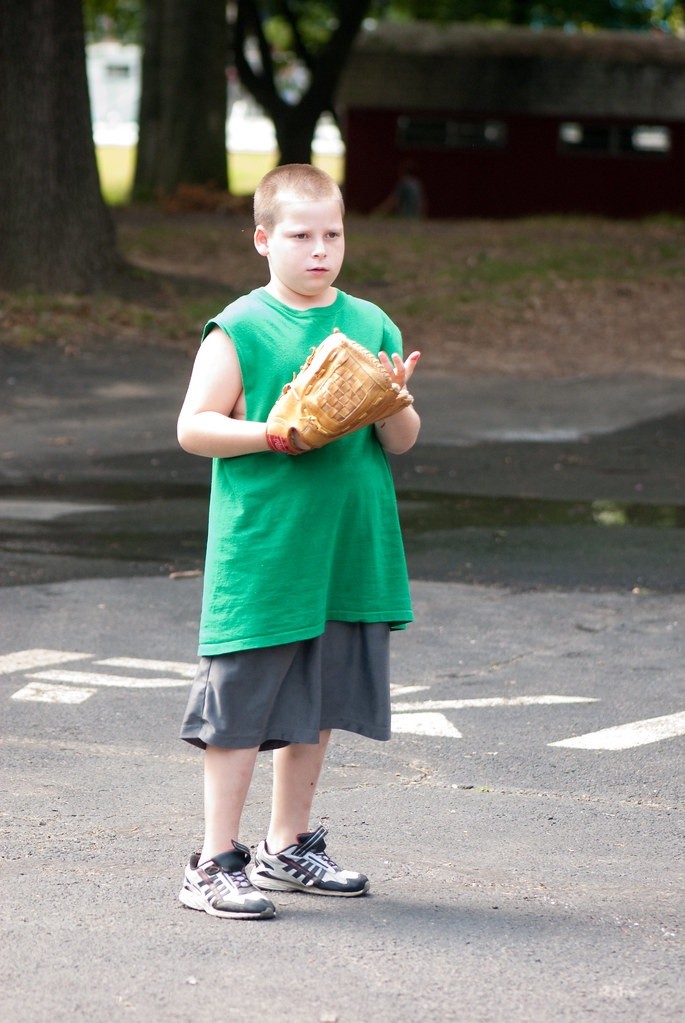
178;839;276;920
251;827;369;897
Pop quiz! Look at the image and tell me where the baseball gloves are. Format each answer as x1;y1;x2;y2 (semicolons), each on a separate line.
265;326;415;457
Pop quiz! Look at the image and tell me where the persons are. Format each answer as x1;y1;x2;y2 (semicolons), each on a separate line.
176;164;420;919
